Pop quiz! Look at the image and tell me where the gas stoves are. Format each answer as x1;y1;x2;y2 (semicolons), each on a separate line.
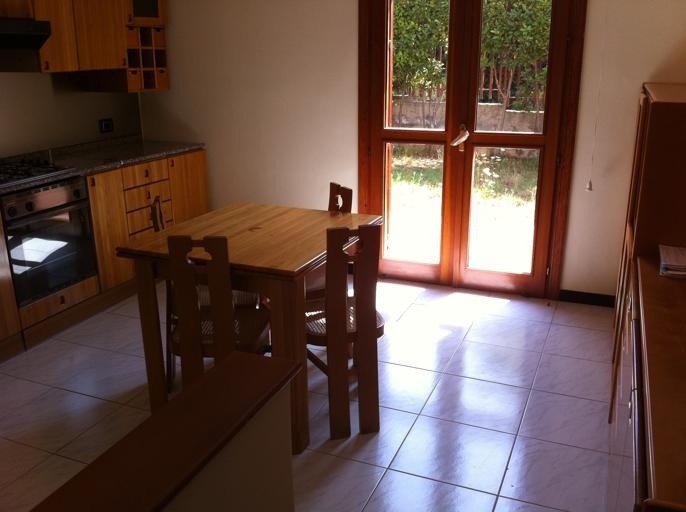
0;157;61;186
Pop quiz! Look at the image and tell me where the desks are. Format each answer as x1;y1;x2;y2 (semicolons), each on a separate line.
608;256;686;512
114;199;386;455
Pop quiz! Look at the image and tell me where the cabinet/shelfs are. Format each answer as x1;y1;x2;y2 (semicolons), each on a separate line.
86;169;135;295
1;215;21;341
32;0;79;74
72;1;128;71
121;158;173;246
167;150;210;227
604;80;686;363
128;0;167;28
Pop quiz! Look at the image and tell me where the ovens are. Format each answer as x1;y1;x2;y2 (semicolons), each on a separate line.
0;176;97;308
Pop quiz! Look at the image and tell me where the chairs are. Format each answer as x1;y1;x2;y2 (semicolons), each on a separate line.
166;236;271;387
303;223;383;442
328;182;354;214
147;190;262;314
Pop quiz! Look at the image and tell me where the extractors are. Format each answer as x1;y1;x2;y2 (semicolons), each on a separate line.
0;18;52;76
